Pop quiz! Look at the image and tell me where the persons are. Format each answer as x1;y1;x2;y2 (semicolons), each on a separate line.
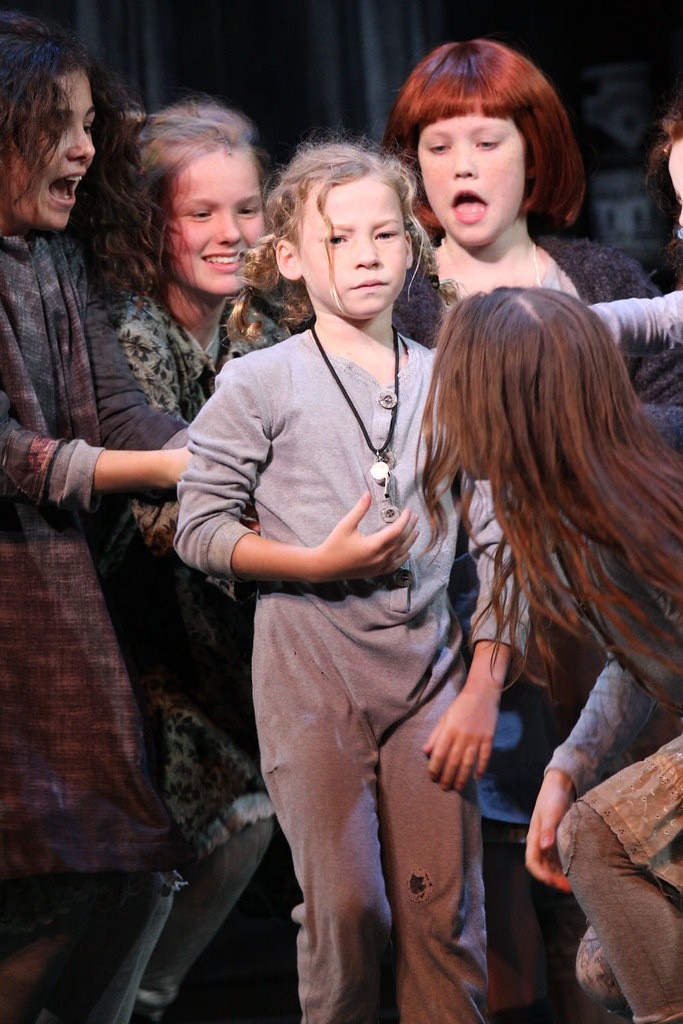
580;100;683;402
417;286;683;1024
0;13;196;1024
174;138;532;1024
289;41;683;1024
84;97;316;1024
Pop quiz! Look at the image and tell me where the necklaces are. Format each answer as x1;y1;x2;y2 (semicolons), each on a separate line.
308;325;399;497
